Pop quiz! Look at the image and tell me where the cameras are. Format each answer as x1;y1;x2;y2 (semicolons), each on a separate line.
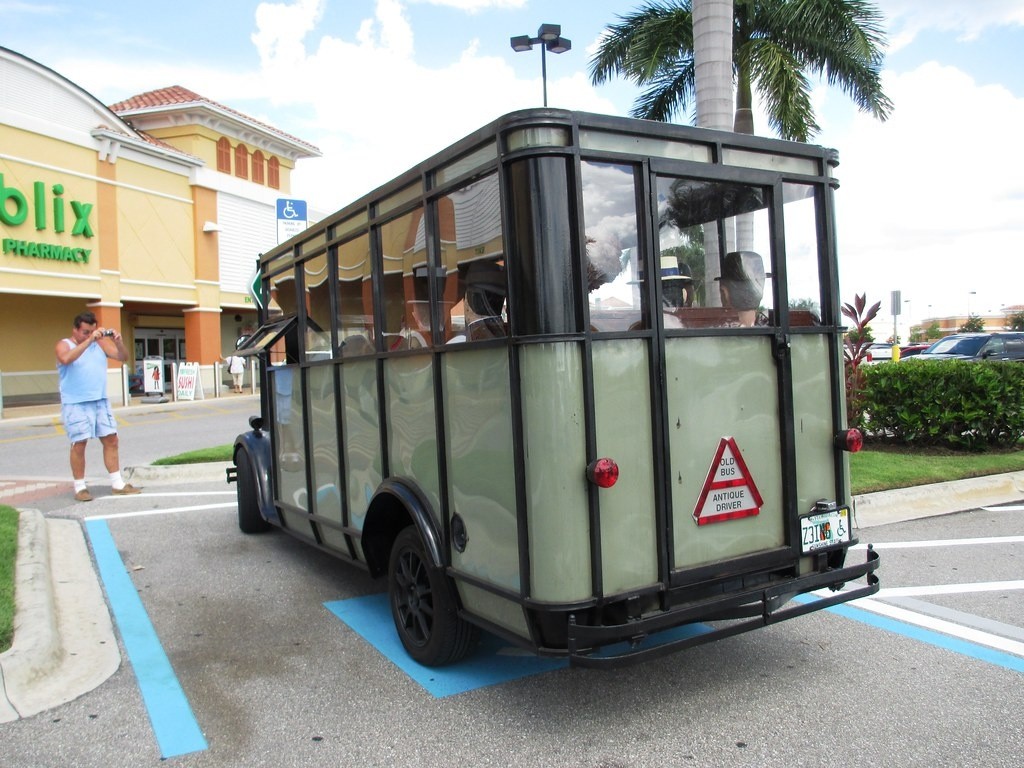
104;330;113;337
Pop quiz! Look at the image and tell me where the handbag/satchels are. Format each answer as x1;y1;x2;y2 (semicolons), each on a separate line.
227;365;232;374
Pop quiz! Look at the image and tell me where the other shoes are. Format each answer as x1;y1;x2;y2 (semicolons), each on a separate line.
73;489;93;501
112;484;142;495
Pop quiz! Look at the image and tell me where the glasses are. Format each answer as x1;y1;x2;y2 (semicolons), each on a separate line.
83;329;94;334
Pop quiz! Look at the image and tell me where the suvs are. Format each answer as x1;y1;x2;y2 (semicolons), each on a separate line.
899;332;1024;364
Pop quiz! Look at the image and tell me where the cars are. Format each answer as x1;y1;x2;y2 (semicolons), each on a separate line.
843;341;933;367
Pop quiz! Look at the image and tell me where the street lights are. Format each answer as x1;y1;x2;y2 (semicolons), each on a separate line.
509;24;572;107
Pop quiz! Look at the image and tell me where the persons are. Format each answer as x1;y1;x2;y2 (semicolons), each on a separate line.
714;251;773;327
152;366;160;389
390;288;455;351
459;262;507;343
55;310;141;501
219;350;247;393
625;255;696;309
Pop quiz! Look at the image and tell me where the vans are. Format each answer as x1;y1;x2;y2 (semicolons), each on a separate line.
226;105;882;670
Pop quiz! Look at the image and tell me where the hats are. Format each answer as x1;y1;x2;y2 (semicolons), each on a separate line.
458;262;507;296
626;256;692;285
406;263;456;304
714;251;775;281
